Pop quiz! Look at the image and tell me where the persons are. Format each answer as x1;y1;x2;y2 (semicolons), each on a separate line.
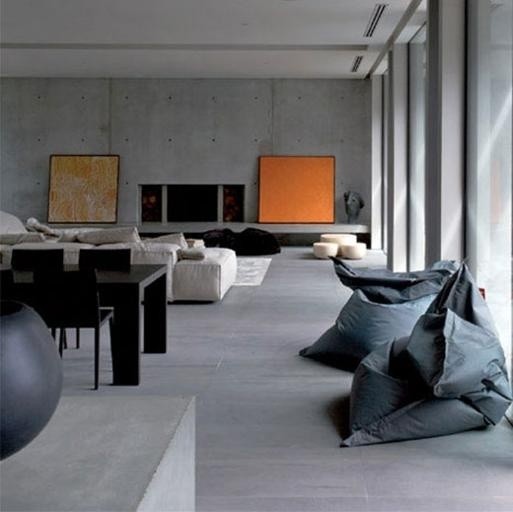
344;191;364;224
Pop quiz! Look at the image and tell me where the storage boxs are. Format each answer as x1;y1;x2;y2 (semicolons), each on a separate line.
173;247;235;303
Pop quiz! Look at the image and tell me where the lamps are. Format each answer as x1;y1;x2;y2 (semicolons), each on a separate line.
79;248;130;265
0;270;15;298
10;249;64;340
32;269;113;390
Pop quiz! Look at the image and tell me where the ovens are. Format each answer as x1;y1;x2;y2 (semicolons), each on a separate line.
0;210;206;304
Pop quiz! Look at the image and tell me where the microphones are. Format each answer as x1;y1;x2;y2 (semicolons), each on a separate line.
341;243;367;260
313;243;338;259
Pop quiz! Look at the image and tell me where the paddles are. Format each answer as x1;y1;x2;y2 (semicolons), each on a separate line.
232;257;272;286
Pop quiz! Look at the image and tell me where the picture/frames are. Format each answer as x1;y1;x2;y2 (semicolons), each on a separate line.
320;234;357;257
0;263;167;384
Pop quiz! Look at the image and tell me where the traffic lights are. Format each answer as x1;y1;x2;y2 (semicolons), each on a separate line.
146;232;188;246
57;228;103;241
77;225;139;245
